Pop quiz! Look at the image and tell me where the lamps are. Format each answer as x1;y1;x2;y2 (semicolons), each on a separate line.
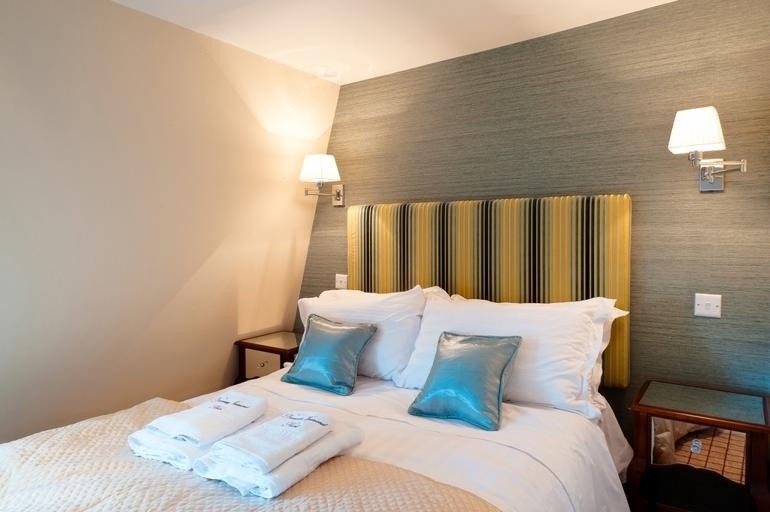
300;153;346;207
667;104;747;195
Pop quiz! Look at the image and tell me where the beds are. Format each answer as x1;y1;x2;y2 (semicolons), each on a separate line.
2;191;632;512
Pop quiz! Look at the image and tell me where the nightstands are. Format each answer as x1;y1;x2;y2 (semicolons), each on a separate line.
622;378;769;510
233;329;306;380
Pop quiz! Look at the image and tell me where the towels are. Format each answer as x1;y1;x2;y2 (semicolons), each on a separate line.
126;390;361;500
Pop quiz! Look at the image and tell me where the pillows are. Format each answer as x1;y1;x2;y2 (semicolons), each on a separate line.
407;333;522;431
396;294;628;419
294;284;446;383
283;313;377;396
